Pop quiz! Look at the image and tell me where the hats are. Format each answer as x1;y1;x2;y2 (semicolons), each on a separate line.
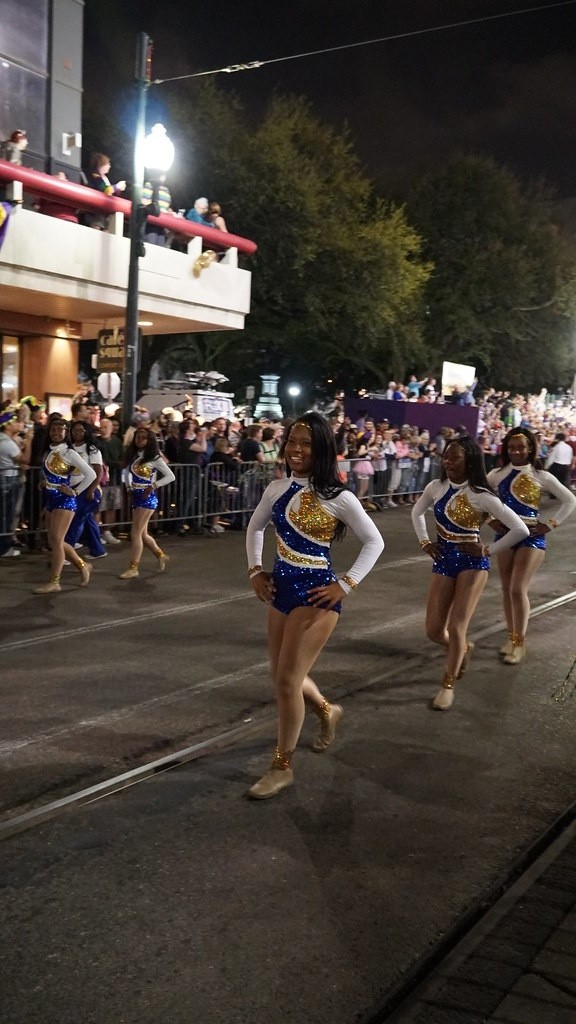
131;411;148;422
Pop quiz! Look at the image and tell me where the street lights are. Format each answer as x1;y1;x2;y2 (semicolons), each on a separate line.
288;383;303;417
119;122;175;443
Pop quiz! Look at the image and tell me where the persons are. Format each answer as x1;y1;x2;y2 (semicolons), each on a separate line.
62;421;107;565
116;407;293;538
442;372;576;499
120;429;175;578
173;196;229;262
329;412;469;513
245;411;385;798
0;130;28;201
34;418;97;593
412;437;531;710
142;174;173;247
386;376;446;405
0;390;122;557
484;427;576;663
34;154;126;230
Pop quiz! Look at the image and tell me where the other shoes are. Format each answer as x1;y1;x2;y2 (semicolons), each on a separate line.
79;563;92;586
61;531;121;566
248;769;294;799
156;554;169;572
119;567;139;579
457;642;474;680
432;685;454;710
356;491;426;513
312;704;343;753
503;646;525;664
1;547;21;557
500;641;513;655
33;581;61;594
210;525;224;533
